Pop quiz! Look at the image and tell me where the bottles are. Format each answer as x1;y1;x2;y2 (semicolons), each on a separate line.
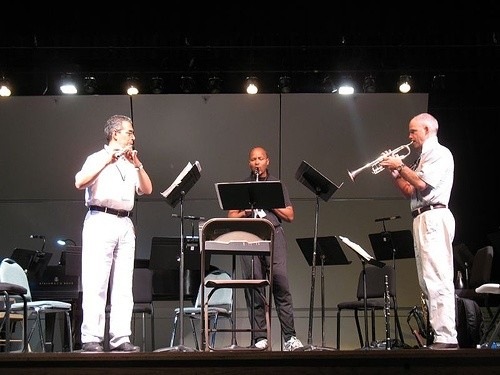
476;342;500;349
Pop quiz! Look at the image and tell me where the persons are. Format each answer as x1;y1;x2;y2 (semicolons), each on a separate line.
227;146;305;350
379;114;462;352
75;115;154;353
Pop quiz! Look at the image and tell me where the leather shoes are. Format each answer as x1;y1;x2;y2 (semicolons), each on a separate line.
82;342;103;352
115;343;140;352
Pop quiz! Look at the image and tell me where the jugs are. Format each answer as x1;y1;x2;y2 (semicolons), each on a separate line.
454;269;464;289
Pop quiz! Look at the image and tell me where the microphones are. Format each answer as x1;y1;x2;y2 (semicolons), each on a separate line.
172;214;205;220
29;235;45;239
375;215;402;222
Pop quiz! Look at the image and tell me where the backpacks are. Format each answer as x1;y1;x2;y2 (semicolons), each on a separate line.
455;296;485;348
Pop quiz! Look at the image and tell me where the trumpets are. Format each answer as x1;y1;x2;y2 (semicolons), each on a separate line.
345;140;414;183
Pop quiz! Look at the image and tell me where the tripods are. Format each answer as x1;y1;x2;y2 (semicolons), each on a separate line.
296;236;352;350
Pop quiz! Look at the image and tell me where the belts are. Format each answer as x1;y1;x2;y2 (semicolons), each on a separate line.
90;206;134;217
411;203;446;218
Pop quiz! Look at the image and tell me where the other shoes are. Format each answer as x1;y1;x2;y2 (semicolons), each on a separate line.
428;343;458;350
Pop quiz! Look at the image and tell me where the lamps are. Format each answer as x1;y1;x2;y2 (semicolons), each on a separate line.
278;76;293;92
397;74;413;93
181;73;196;94
320;74;376;94
125;74;140;95
0;72;14;97
84;75;98;94
432;72;448;90
208;74;225;92
151;75;166;94
245;72;260;94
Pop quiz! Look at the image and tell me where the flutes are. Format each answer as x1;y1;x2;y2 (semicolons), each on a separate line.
116;144;134;159
383;275;391;350
251;167;264;219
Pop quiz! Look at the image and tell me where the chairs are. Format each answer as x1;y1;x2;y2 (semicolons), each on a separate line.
106;268;156;352
0;257;73;353
200;217;276;351
475;282;500;349
455;246;493;321
337;267;404;350
171;270;233;351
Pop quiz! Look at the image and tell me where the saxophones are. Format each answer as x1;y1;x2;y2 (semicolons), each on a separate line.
407;292;434;339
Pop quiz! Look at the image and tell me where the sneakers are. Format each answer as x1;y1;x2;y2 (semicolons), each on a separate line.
255;339;267;349
284;336;304;351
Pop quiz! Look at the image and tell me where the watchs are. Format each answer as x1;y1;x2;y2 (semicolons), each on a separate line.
135;164;144;171
397;163;405;172
